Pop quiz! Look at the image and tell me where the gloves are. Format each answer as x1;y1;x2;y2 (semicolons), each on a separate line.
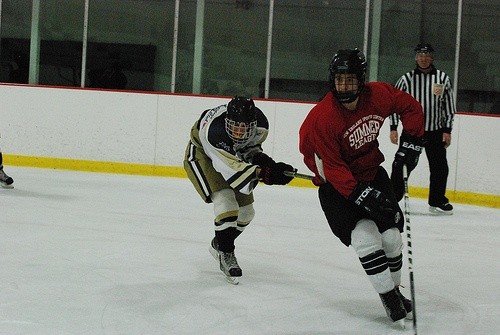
252;152;297;185
391;136;421;176
348;182;393;217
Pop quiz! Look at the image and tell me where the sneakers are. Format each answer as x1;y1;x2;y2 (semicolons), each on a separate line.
0;165;14;188
209;237;242;285
428;195;454;215
379;285;413;329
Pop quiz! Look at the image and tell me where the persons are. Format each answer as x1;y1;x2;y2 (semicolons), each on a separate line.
389;43;455;216
298;48;425;328
183;96;298;284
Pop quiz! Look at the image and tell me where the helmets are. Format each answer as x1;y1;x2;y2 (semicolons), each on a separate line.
224;97;257;149
328;47;368;103
414;42;434;56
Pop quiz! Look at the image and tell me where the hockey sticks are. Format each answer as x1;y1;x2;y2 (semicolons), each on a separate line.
283;171;317;181
402;164;417;335
